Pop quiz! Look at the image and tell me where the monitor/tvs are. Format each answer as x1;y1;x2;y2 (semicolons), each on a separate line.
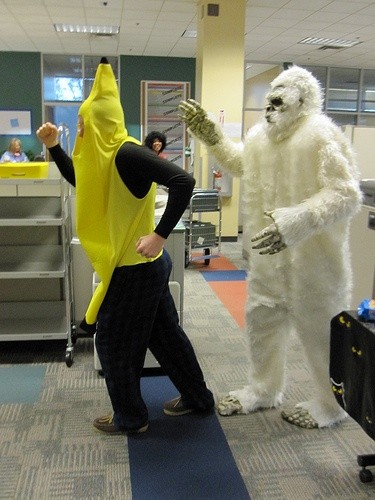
43;76;84;101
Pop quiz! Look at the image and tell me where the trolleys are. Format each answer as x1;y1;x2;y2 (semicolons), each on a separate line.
0;178;77;367
185;188;223;267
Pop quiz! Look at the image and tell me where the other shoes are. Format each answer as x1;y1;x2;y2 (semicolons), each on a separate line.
94;414;148;432
162;396;194;416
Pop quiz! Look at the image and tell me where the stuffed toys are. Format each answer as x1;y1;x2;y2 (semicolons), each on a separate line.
178;64;364;426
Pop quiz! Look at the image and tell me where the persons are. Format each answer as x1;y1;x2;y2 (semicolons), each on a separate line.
145;130;168;159
36;102;216;435
1;138;30;163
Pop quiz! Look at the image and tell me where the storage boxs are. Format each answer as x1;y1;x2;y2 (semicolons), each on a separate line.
0;161;50;180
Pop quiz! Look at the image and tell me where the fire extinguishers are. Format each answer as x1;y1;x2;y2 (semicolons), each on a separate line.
213;171;223;190
